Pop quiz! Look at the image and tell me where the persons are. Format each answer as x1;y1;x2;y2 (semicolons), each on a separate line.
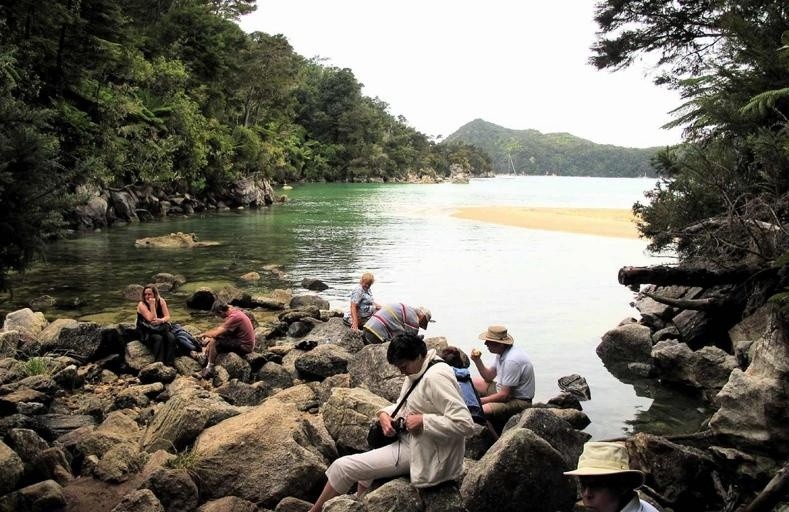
562;440;660;512
193;297;256;381
136;284;175;367
350;272;382;332
362;301;431;346
307;331;474;512
440;345;487;428
468;325;537;425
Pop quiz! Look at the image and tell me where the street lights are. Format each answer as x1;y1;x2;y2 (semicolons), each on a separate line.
367;420;399;451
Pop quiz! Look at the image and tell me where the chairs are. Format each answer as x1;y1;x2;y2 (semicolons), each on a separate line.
190;350;216;379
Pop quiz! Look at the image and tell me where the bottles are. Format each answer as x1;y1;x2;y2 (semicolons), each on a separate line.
560;439;647;490
478;326;514;345
442;345;471;369
420;307;431;330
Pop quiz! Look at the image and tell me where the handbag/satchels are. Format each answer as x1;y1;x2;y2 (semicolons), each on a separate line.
162;318;165;323
